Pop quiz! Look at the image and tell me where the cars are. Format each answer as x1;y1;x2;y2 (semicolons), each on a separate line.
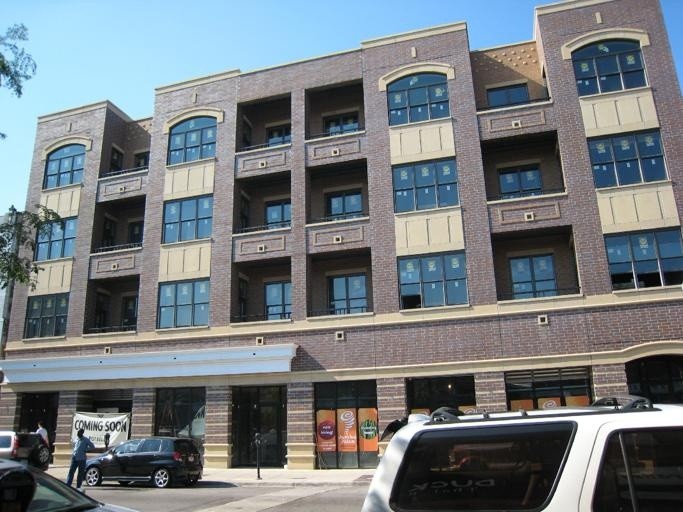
82;435;204;489
0;457;143;512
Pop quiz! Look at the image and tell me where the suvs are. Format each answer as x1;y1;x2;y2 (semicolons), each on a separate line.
0;430;52;473
356;392;683;512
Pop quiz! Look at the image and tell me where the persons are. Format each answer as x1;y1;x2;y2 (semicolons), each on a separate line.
35;421;48;447
65;428;94;489
